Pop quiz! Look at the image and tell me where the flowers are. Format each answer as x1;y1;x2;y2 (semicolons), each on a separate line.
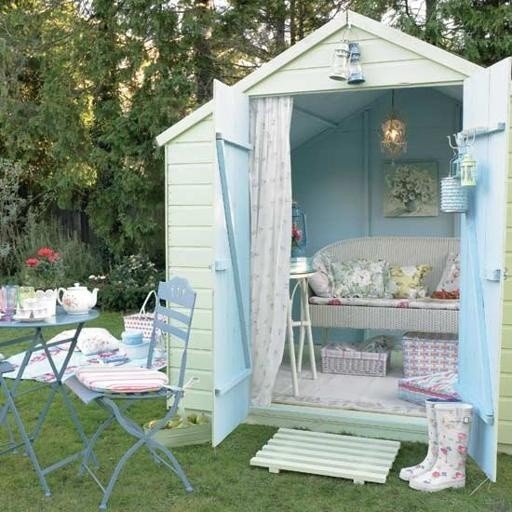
386;164;435;203
291;223;303;257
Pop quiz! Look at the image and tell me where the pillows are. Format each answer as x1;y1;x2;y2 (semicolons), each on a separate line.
443;253;460;297
384;262;433;300
434;253;460;298
328;257;386;299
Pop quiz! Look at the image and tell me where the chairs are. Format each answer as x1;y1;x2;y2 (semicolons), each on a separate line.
63;276;196;509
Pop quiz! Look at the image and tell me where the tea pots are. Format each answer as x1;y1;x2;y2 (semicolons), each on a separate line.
57;282;100;315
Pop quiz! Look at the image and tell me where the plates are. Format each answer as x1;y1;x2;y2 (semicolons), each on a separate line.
14;318;48;322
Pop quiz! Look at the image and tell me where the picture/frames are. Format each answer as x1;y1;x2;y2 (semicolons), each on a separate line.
383;160;440;217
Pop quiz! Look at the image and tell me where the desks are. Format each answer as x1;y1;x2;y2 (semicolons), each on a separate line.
0;309;98;496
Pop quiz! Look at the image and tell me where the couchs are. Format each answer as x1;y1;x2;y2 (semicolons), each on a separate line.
297;235;463;372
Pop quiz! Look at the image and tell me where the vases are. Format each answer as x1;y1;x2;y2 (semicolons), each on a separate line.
289;258;315;274
404;200;418;211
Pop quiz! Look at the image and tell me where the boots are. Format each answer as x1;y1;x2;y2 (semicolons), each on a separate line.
400;399;461;481
410;405;473;493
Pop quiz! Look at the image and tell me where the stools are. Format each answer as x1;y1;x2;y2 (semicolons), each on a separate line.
279;269;320;397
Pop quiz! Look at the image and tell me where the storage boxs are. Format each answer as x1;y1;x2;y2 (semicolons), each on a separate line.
400;333;459;377
320;342;390;377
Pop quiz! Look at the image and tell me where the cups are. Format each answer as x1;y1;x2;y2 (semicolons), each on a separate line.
0;286;17;321
19;286;35;308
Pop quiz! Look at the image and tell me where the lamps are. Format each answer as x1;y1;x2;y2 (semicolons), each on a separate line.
329;28;366;85
379;89;408;155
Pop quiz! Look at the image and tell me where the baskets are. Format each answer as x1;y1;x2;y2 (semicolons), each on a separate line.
122;291;166;344
